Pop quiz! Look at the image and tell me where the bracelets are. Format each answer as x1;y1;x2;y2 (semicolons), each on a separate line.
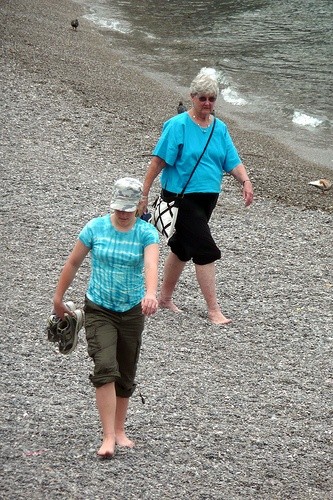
242;180;251;185
140;195;148;199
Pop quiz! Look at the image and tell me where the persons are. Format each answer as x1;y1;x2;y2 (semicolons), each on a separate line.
54;177;161;457
135;67;254;325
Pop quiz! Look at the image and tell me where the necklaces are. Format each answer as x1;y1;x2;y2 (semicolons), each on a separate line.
192;108;211;133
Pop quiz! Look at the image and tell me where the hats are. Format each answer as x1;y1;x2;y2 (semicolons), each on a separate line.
109;178;143;213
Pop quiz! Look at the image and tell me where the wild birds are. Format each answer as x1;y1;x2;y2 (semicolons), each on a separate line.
70;19;79;30
307;178;332;195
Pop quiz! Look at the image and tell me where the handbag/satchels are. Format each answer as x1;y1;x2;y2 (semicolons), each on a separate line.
148;196;179;239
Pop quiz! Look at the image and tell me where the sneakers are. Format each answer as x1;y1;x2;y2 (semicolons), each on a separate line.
57;308;85;355
47;301;75;342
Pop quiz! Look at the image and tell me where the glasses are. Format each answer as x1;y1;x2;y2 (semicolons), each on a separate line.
198;96;216;102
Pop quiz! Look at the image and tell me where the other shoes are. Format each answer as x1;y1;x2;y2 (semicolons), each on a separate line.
140;213;153;224
